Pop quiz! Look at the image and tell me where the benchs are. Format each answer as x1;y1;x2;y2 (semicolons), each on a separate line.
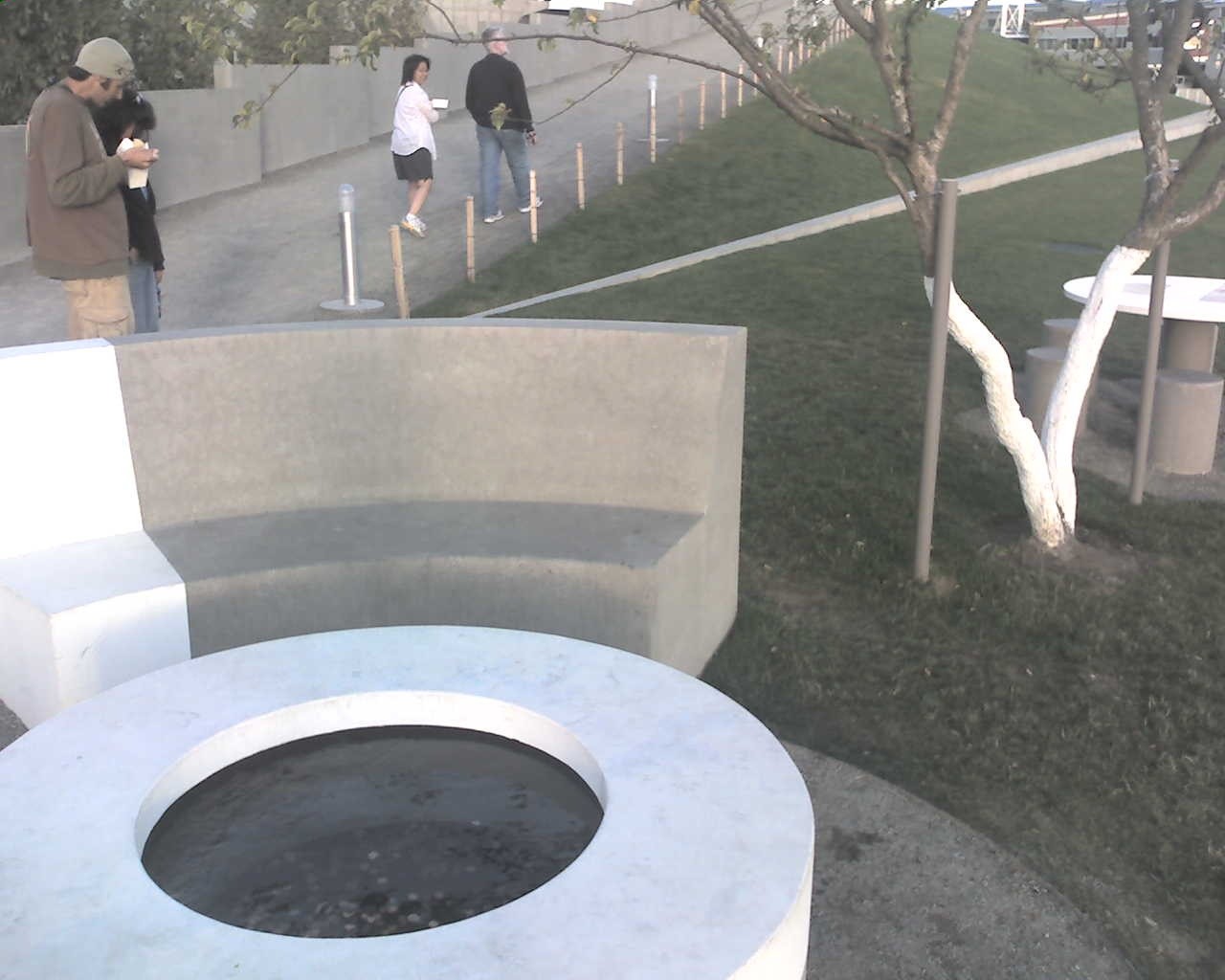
0;317;748;733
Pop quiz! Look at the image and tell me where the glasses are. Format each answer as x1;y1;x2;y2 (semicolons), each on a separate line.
133;93;143;105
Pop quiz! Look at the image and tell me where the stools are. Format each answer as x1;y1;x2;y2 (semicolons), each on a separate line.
1026;346;1098;436
1043;318;1079;346
1151;369;1224;475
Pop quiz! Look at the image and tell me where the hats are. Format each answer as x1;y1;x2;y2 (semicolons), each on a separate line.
75;37;134;80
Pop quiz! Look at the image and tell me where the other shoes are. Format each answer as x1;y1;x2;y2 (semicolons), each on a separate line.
401;216;424;238
484;210;504;222
520;197;543;212
420;221;426;231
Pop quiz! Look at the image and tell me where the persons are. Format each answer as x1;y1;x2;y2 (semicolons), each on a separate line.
87;88;165;335
465;27;543;223
390;53;438;239
23;38;159;341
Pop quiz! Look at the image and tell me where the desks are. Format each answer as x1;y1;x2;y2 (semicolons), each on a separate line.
1062;275;1225;373
0;625;816;980
